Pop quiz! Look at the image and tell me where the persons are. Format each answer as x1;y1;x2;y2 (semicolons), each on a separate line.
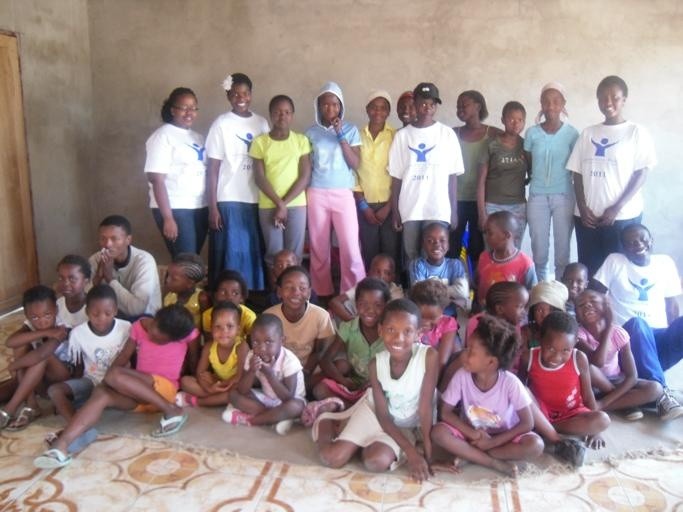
475;100;530;250
305;82;365;303
523;82;582;282
387;79;467;268
449;89;502;283
1;211;683;482
143;86;210;257
397;91;418;125
355;89;397;270
563;75;651;293
202;72;273;292
249;92;311;280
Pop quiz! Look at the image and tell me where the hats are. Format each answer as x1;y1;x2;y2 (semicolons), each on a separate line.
397;91;415;112
414;82;442;105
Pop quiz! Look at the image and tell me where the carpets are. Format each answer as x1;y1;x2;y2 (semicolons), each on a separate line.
73;362;683;485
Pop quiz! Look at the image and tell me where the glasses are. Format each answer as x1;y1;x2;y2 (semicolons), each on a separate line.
171;103;200;114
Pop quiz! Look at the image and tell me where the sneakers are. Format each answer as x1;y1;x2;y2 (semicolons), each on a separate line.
175;392;199;408
271;419;294;437
67;428;98;454
656;388;683;421
221;407;254;429
553;437;587;467
624;406;645;422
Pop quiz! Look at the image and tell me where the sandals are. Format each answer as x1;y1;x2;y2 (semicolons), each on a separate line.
1;407;18;431
6;406;42;432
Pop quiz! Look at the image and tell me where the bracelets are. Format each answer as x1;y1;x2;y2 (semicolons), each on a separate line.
337;133;345;138
358;198;368;210
338;135;347;141
340;139;348;144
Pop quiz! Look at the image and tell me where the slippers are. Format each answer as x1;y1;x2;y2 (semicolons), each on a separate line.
301;397;345;428
33;448;73;471
43;429;61;447
150;410;188;438
